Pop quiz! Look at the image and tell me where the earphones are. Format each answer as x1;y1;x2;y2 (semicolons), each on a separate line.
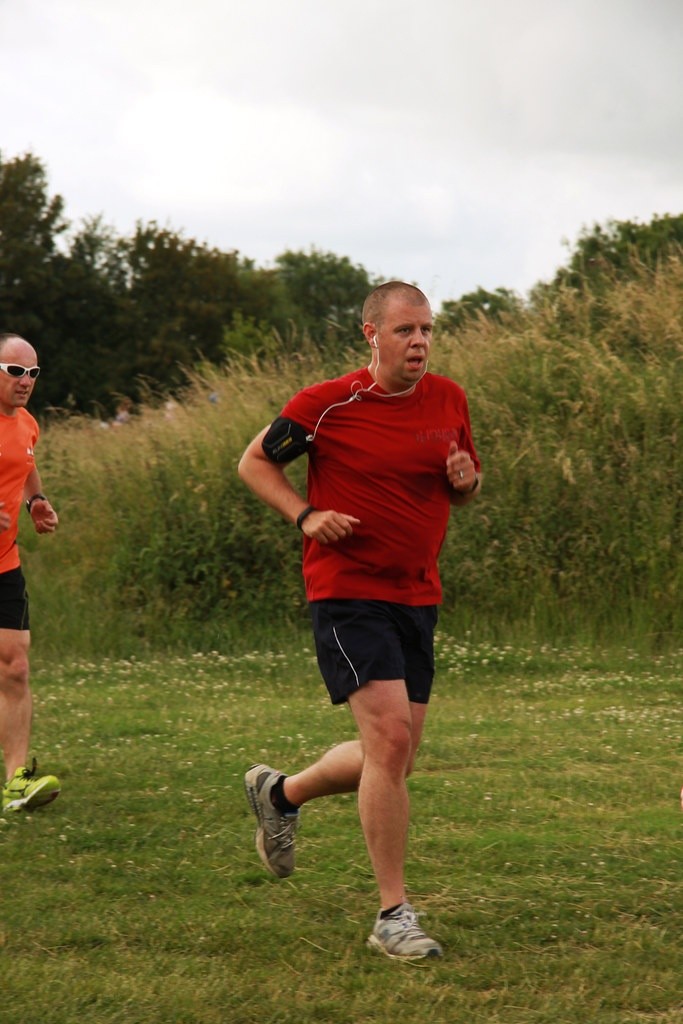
373;335;378;348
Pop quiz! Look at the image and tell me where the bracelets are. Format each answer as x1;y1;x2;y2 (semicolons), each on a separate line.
296;506;314;532
25;495;46;513
471;474;478;492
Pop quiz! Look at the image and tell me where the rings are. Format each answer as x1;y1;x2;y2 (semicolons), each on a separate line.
460;470;463;478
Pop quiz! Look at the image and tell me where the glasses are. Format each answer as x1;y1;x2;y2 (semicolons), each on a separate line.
0;364;40;378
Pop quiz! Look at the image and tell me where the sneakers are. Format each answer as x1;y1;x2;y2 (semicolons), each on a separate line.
2;756;60;812
243;763;299;879
366;903;442;960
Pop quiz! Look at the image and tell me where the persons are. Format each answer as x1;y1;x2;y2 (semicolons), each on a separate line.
0;332;61;814
236;281;482;960
